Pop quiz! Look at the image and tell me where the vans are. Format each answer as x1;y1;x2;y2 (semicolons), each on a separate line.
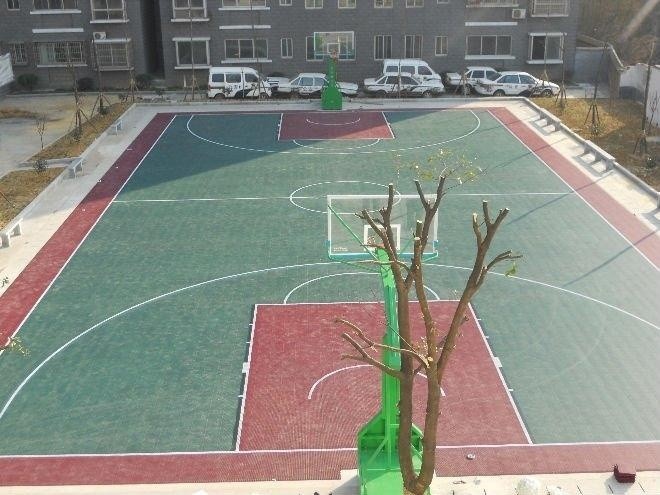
383;58;444;93
206;66;273;99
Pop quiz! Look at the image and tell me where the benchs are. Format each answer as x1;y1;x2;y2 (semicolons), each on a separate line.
0;216;22;246
540;107;560;130
656;192;660;209
111;119;123;135
67;157;84;179
584;138;616;172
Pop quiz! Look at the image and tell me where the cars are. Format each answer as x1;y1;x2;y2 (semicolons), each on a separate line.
474;71;560;97
277;72;359;99
363;72;441;98
446;66;499;94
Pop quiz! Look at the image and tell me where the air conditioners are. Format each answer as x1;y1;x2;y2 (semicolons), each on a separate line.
93;32;107;41
511;9;526;20
